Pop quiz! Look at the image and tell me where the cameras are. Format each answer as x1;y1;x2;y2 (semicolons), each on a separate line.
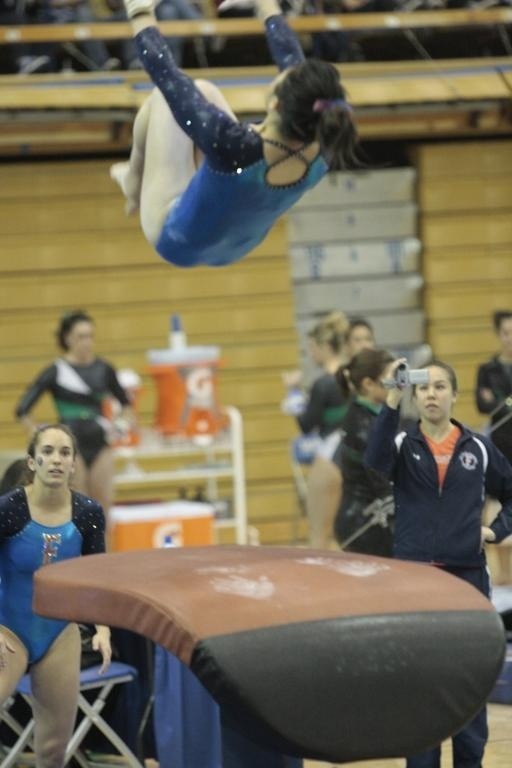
382;363;429;389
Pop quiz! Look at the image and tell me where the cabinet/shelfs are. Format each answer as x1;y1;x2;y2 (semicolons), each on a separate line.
92;406;249;551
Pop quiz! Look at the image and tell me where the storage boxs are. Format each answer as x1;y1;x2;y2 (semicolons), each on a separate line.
110;498;215;551
144;346;229;436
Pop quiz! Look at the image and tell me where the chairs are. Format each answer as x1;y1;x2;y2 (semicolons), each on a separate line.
0;662;144;767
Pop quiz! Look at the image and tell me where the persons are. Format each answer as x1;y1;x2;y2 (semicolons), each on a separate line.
15;313;130;524
0;424;112;767
366;357;512;768
476;311;512;542
336;349;397;560
107;0;369;269
284;312;376;546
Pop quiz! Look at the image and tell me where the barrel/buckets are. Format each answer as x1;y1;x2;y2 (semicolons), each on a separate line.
143;356;232;440
